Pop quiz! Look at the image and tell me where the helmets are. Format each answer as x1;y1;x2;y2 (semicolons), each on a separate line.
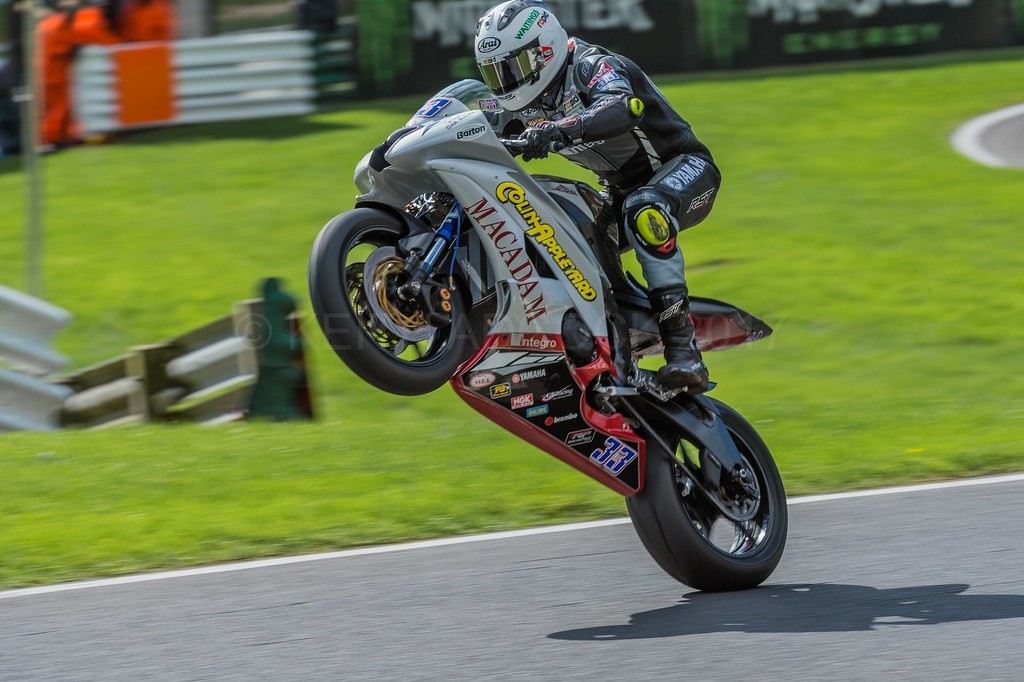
471;0;567;112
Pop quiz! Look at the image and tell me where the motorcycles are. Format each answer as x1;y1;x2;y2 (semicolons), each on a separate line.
302;76;790;594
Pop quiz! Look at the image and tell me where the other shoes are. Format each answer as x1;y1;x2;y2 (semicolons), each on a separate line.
34;139;57;154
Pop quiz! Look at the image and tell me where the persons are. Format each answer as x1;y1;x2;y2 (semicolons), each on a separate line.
30;1;178;155
470;0;721;386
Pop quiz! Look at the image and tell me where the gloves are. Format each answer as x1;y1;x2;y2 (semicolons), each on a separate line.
517;118;582;163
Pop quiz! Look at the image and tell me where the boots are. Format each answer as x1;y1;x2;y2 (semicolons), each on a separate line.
649;285;710;389
